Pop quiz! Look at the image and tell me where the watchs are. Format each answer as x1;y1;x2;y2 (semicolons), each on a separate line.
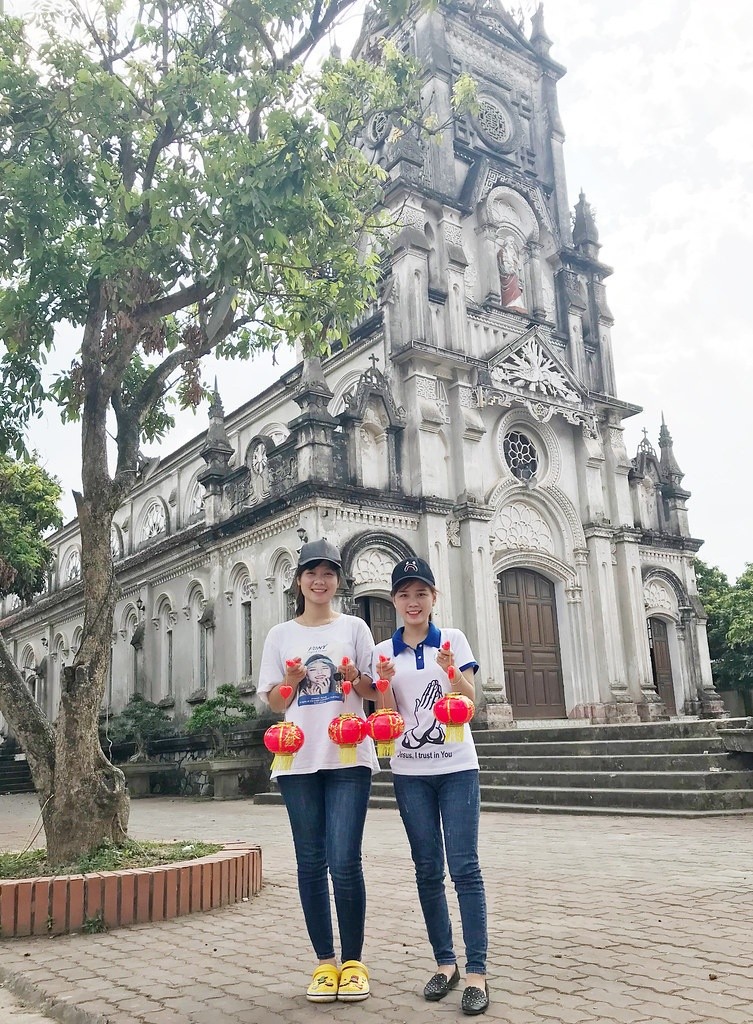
351;667;361;686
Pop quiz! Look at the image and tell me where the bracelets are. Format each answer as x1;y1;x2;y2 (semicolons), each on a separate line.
451;675;464;686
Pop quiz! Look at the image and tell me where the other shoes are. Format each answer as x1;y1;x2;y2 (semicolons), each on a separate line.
424;963;460;1001
462;979;489;1014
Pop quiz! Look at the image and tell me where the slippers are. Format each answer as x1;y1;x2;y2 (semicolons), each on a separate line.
337;960;370;1002
306;964;340;1002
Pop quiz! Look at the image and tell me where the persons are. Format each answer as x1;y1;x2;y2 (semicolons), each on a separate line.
370;558;490;1016
498;235;527;308
257;538;381;1001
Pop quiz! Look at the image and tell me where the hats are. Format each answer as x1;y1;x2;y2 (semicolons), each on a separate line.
298;539;341;568
392;558;435;586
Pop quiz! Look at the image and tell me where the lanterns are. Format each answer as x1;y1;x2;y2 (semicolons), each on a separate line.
264;720;305;772
328;712;367;763
434;692;476;742
366;707;405;756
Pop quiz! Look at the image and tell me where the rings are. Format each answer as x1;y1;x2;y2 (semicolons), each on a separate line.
347;669;350;672
441;656;446;660
